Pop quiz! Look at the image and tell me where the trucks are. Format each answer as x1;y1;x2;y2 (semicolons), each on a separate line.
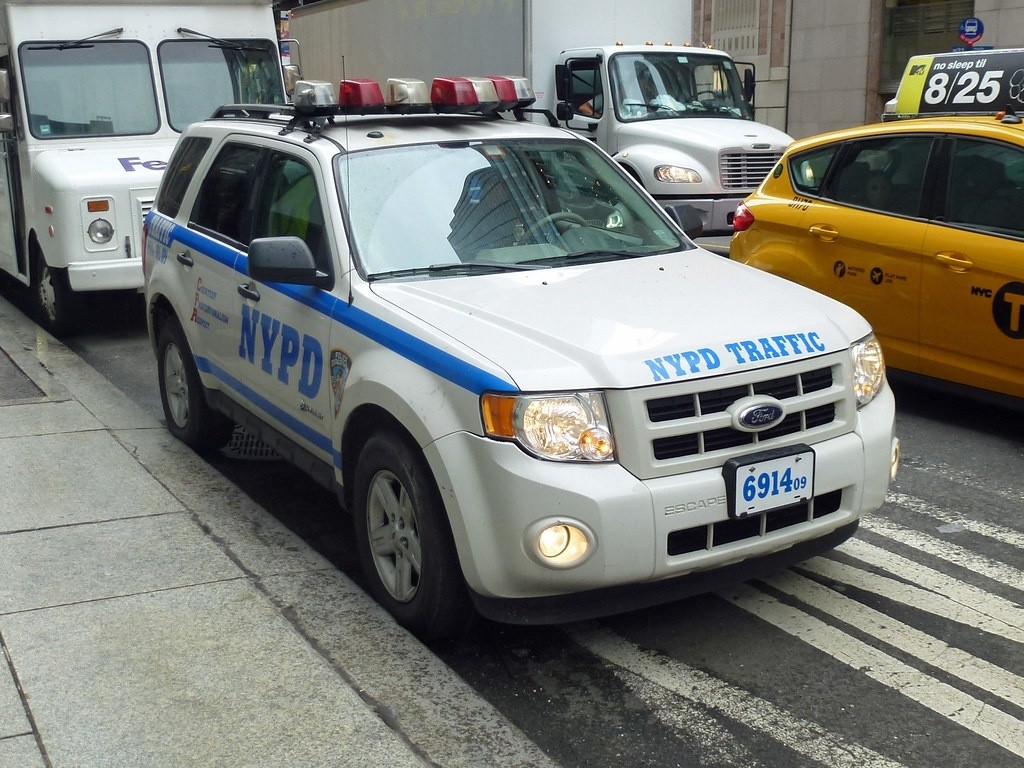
0;0;314;331
283;0;814;247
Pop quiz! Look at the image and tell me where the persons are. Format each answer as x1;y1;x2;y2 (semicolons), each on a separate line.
847;168;905;214
266;161;383;252
580;81;635;118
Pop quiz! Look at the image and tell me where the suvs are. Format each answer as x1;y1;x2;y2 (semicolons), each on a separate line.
138;71;903;635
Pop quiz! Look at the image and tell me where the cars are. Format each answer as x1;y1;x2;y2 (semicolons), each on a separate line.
726;49;1024;397
